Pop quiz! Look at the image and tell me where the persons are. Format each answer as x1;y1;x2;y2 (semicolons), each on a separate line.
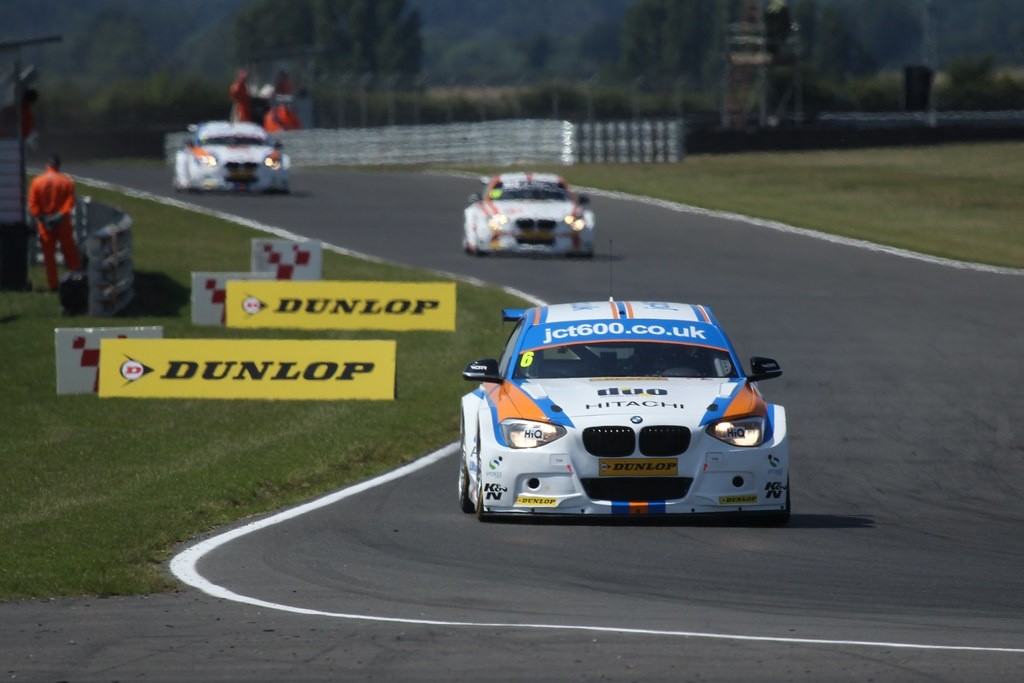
263;71;302;133
627;344;696;378
27;153;79;291
229;70;253;122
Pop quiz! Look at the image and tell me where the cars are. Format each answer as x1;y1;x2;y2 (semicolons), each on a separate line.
172;120;293;198
459;302;790;528
463;171;597;259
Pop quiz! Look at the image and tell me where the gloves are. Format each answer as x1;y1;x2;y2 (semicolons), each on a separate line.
39;211;62;232
